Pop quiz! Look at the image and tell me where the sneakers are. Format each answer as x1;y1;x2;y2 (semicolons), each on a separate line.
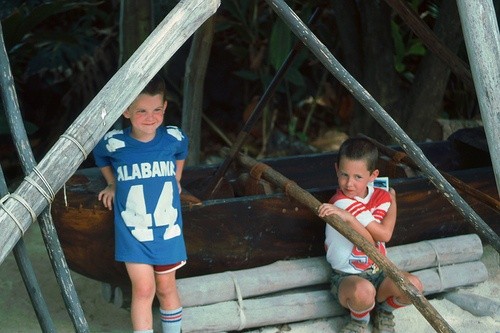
373;307;397;333
339;319;367;333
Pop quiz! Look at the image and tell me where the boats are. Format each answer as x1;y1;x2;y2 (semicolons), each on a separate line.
49;125;499;298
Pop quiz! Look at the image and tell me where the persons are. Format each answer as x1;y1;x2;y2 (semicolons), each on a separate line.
316;135;422;333
92;81;190;333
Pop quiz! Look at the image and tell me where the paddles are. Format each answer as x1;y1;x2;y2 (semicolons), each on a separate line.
187;2;328;201
